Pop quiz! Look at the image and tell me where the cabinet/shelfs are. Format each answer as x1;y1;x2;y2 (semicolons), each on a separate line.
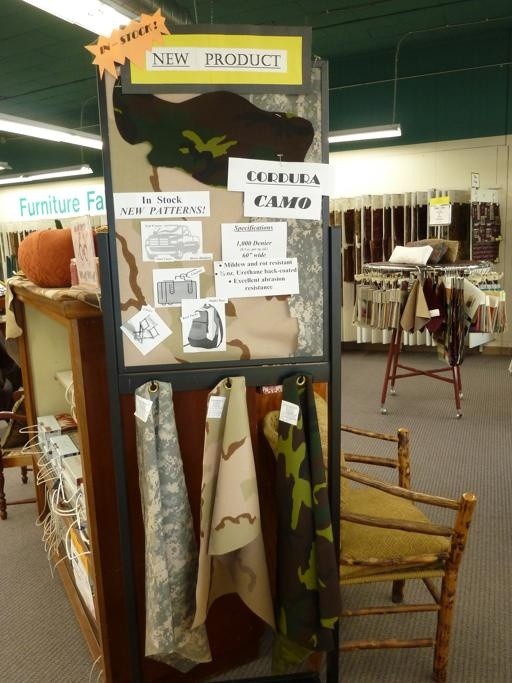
362;262;497;418
5;279;132;683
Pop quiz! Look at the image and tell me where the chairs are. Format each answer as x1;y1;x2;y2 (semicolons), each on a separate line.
264;405;478;683
0;411;32;520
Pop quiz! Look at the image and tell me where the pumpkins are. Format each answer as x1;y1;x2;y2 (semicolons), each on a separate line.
16;219;75;288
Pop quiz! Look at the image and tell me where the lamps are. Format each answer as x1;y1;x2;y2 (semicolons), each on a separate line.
327;32;402;142
0;113;104;150
0;105;94;185
23;0;196;36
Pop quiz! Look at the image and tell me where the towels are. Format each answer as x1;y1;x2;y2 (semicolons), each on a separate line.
188;376;275;659
137;374;214;675
274;375;341;652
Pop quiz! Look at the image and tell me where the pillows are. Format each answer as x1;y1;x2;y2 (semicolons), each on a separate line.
388;238;460;267
0;395;29;448
